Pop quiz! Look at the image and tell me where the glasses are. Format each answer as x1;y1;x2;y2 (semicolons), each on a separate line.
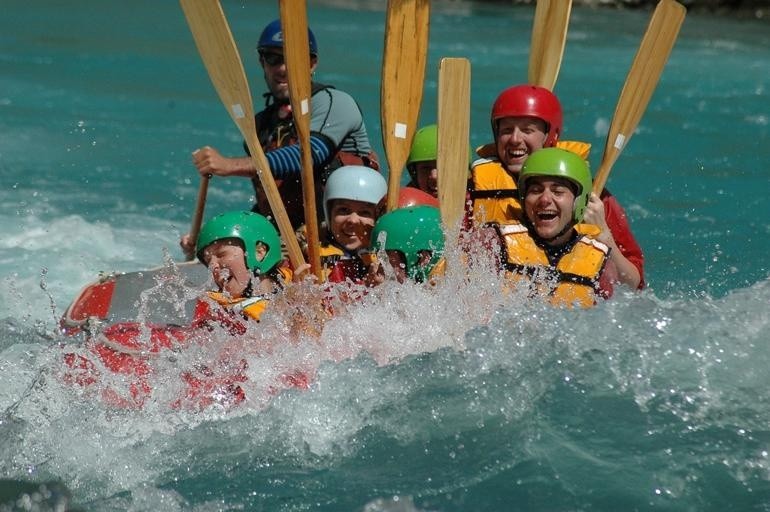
257;50;285;65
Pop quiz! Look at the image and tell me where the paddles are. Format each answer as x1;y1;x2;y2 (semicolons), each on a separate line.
528;0;574;91
437;56;470;281
378;0;429;209
280;0;321;282
588;0;689;208
179;0;313;280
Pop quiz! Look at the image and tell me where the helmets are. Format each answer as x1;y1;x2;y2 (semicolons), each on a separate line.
323;124;472;281
519;146;594;222
257;18;317;57
491;86;564;149
195;209;281;275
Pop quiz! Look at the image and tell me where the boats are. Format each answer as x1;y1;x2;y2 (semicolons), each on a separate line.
61;259;312;422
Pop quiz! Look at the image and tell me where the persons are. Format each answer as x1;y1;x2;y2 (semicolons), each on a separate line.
288;167;386;299
402;123;486;243
467;148;618;318
181;19;380;261
365;202;477;292
469;84;644;294
188;208;297;329
